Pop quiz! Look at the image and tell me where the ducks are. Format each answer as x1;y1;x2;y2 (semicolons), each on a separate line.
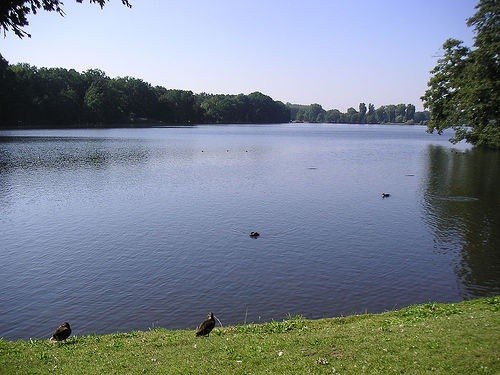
51;322;72;343
382;193;390;198
250;232;259;236
196;312;215;337
